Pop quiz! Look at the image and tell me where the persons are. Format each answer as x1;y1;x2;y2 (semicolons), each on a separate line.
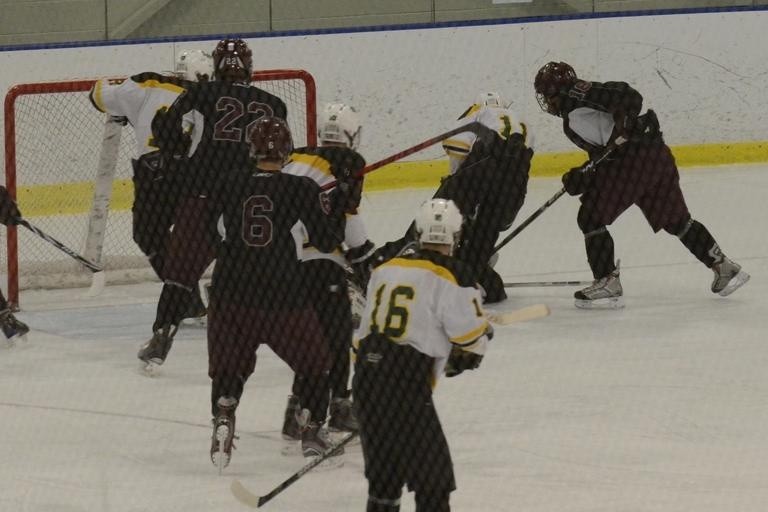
351;197;494;511
0;183;29;348
88;36;376;470
534;61;751;312
371;91;533;305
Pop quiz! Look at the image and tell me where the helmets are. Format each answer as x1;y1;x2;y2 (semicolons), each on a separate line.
534;61;577;99
415;198;464;257
174;50;216;83
250;117;293;158
211;38;253;83
473;90;502;107
318;103;361;151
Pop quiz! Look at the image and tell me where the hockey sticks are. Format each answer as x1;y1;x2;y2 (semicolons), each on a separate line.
488;134;629;267
232;428;359;507
319;122;497;192
21;219;106;298
484;304;550;324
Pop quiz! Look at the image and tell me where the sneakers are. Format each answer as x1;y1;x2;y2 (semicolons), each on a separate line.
210;394;239;468
708;243;741;293
138;324;179;365
282;393;361;458
0;308;29;339
182;282;207;318
574;259;623;300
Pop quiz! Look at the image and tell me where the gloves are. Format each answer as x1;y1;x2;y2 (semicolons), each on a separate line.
561;160;597;196
0;185;21;225
444;347;483;378
612;106;646;144
347;239;384;290
